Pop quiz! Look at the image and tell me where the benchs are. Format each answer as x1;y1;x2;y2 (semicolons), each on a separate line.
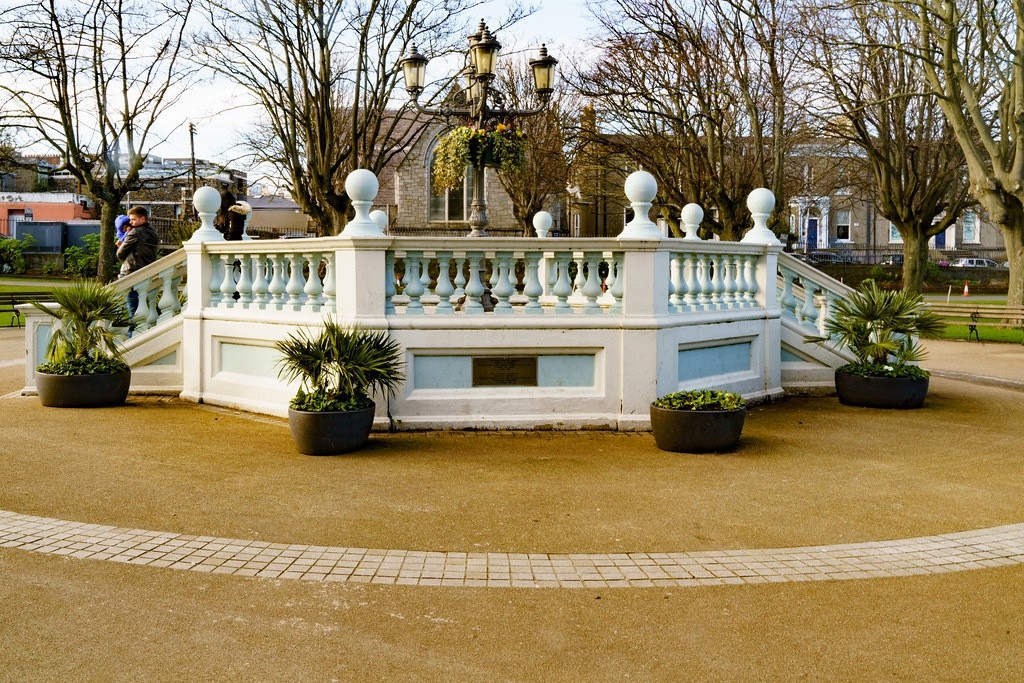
918;303;1024;342
0;291;58;329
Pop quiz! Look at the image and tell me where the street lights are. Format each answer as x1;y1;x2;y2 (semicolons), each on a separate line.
395;16;559;312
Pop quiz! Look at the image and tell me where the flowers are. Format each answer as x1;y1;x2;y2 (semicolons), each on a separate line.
432;123;528;197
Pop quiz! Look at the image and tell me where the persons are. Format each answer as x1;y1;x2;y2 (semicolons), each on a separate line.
115;215;132;242
783;233;797;253
214;191;252;302
116;206;158;339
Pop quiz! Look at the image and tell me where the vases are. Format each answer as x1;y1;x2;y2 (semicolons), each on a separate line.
470;136;503;167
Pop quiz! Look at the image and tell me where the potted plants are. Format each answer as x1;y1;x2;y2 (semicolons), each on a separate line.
28;276;148;408
650;388;748;452
802;279;949;409
273;312;408;455
